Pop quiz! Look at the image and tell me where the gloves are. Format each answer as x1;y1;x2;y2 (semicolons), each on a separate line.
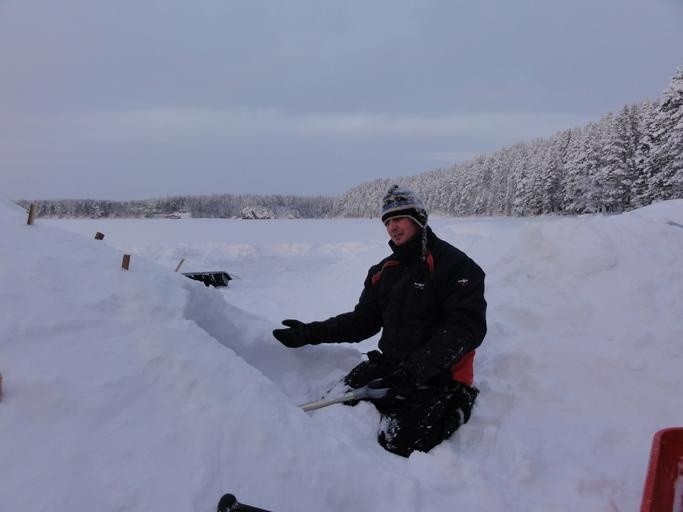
272;318;316;349
367;380;417;407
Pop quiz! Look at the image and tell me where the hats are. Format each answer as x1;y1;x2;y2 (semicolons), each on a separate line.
379;184;428;228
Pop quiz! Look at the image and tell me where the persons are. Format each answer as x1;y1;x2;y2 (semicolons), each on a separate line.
270;182;489;458
